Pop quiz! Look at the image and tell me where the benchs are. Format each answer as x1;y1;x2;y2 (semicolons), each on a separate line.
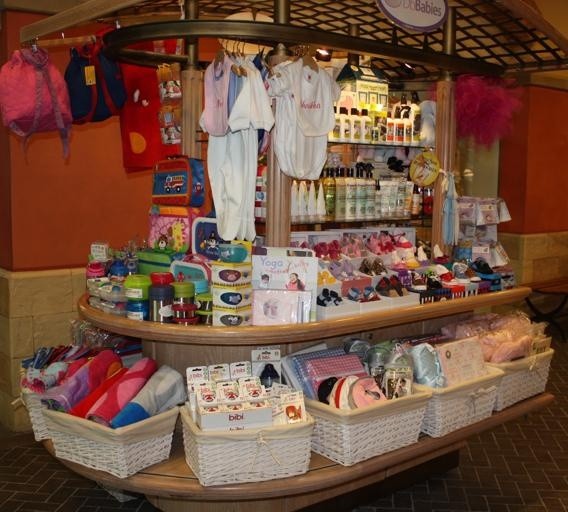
519;276;568;342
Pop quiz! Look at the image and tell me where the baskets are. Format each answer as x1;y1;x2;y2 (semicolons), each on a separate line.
411;345;506;441
177;400;317;486
43;400;179;480
305;381;433;467
18;361;50;443
482;347;555;414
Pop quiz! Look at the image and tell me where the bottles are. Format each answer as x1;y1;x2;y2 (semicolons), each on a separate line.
323;168;382;220
291;181;325;216
328;106;379;144
149;278;213;325
86;260;126;279
411;185;420;218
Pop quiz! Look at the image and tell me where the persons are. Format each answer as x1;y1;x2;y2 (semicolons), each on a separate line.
284;259;309;291
258;273;271;289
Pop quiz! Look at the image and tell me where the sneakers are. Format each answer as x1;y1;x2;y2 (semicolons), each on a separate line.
346;287;381;303
375;274;409;299
316;288;344;309
470;257;503;280
451;260;482;283
298;227;458;287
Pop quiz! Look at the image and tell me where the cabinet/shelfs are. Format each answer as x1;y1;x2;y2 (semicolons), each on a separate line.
19;0;568;512
